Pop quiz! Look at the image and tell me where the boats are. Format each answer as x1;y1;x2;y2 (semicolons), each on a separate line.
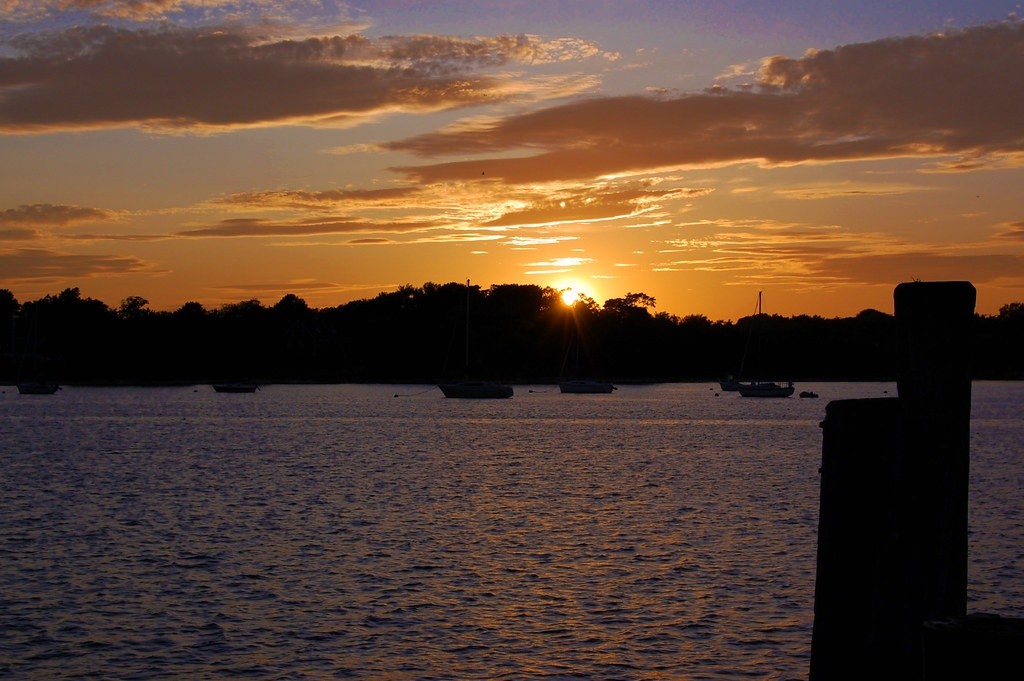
212;383;261;393
15;382;64;395
558;378;618;393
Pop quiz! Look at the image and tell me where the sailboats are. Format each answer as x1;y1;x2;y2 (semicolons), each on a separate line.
718;291;795;398
438;279;513;398
